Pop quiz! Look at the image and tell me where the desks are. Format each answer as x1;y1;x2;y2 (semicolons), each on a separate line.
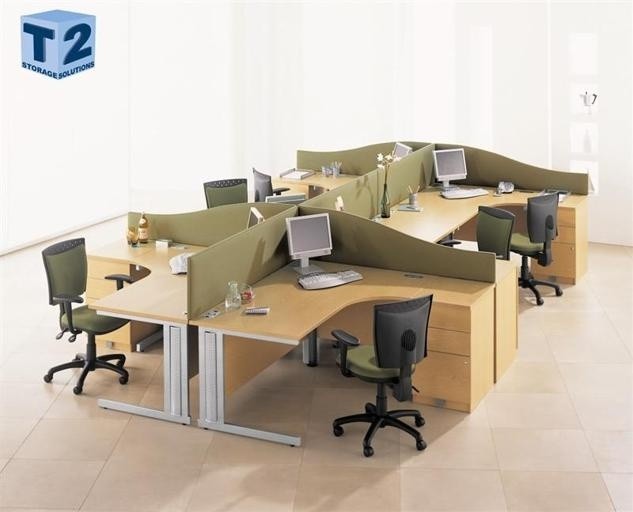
187;259;496;447
85;238;208;425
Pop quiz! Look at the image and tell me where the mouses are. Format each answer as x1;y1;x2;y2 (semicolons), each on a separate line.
496;187;502;195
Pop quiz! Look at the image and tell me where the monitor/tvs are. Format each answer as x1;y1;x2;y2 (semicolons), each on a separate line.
283;212;333;275
265;193;306;204
431;148;467;190
246;206;266;229
393;142;413;157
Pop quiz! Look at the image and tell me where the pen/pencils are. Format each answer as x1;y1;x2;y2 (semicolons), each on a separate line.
407;184;420;194
332;160;342;168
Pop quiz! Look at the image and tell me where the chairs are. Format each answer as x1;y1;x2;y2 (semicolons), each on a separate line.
203;168;290;209
330;294;433;457
41;238;129;395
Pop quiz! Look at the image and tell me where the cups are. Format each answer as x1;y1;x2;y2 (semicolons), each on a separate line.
408;193;417;204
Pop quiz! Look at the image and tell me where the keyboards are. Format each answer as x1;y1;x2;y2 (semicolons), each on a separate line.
439;188;490;199
169;251;194;275
297;269;363;290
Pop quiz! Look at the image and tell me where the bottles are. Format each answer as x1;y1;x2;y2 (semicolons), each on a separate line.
224;280;240;308
380;184;390;217
138;211;147;243
